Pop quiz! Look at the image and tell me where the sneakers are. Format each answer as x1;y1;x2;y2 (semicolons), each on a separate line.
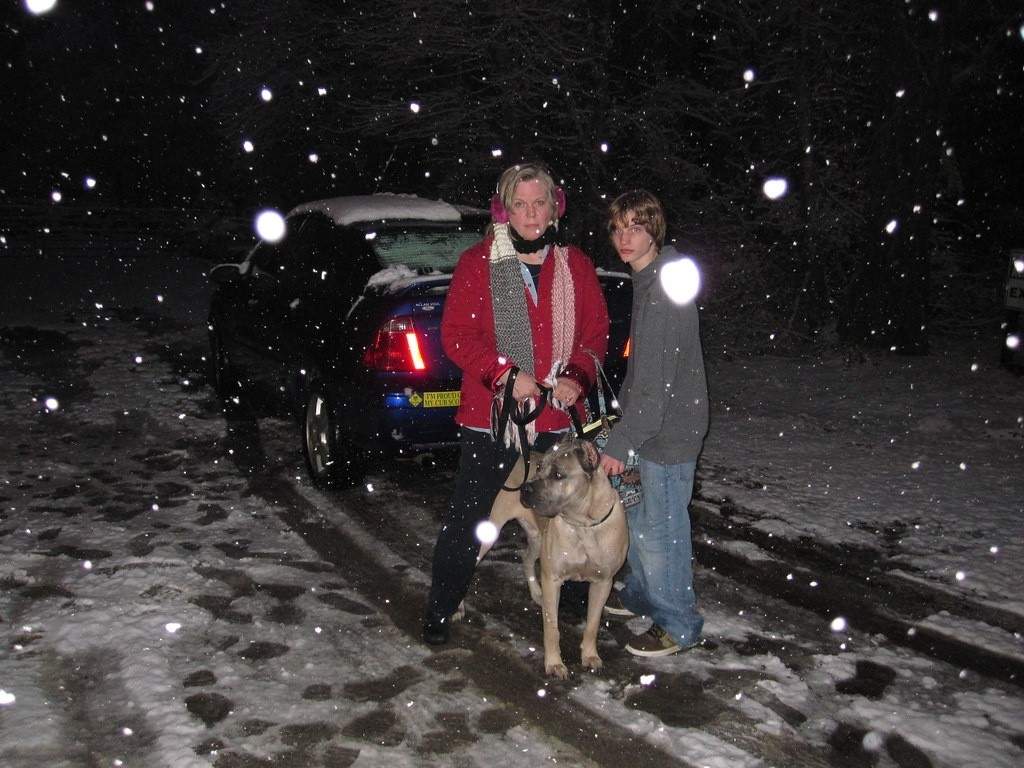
625;623;700;657
603;591;637;616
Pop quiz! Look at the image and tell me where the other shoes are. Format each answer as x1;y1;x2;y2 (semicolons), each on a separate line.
422;606;449;645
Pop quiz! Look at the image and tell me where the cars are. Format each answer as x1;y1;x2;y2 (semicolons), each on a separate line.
206;193;635;489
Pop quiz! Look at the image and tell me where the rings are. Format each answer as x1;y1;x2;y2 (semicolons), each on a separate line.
565;396;570;402
523;398;529;403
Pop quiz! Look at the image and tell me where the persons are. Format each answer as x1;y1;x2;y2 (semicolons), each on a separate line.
420;162;609;647
599;188;710;657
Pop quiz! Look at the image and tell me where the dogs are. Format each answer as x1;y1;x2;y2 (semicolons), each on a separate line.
448;437;632;683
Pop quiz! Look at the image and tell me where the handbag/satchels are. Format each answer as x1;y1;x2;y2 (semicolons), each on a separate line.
581;412;645;509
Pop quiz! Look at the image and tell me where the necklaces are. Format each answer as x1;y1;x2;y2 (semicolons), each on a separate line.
531;272;541;278
